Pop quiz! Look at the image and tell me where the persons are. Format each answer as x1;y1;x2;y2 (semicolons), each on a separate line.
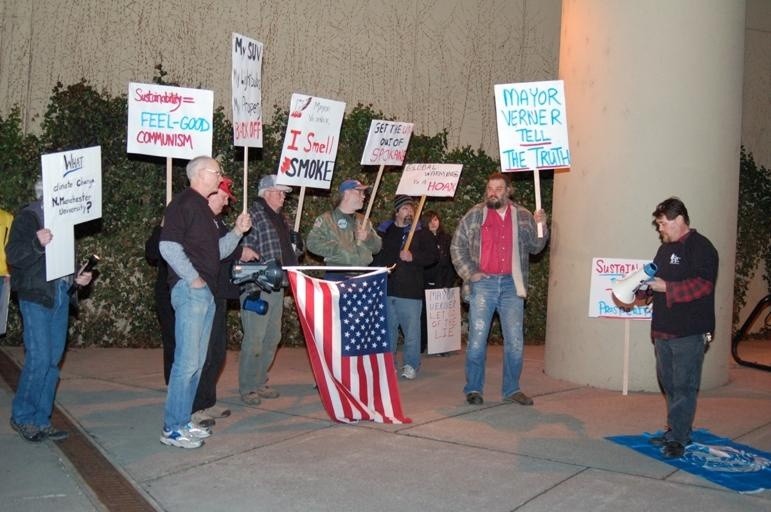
3;175;103;445
305;177;385;284
0;207;15;344
237;173;307;407
157;155;254;450
372;193;440;381
636;197;720;459
144;213;177;387
286;269;415;425
189;176;262;429
419;209;465;359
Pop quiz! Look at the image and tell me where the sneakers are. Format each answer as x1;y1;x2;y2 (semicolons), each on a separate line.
206;405;232;420
467;390;484;405
504;388;533;404
258;384;281;400
184;421;212;439
662;441;686;459
239;390;263;405
159;425;205;450
650;434;693;445
402;365;418;380
10;417;69;442
190;406;216;427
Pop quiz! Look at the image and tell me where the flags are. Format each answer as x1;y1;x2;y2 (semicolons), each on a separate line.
448;173;552;407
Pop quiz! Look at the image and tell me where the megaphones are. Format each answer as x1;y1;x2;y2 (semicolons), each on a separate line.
611;263;658;304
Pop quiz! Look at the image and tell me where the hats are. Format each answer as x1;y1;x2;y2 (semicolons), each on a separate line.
394;194;412;210
219;176;238;203
257;174;292;194
338;181;368;190
